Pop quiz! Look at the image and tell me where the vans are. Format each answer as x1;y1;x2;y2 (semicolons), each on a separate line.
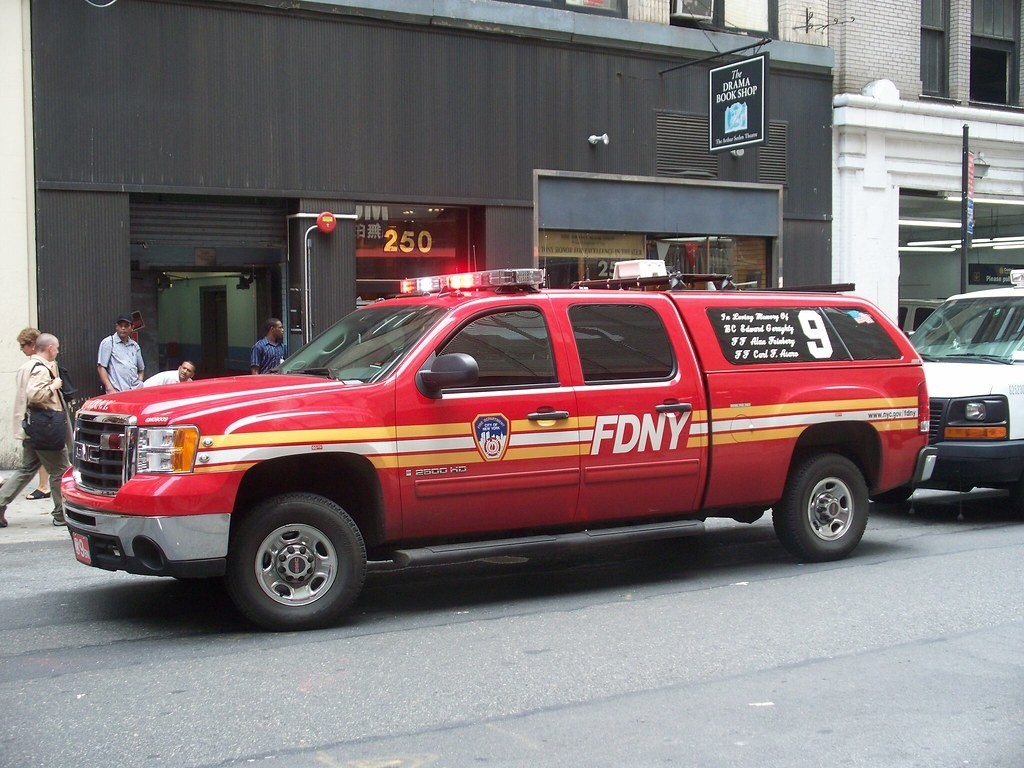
869;270;1024;509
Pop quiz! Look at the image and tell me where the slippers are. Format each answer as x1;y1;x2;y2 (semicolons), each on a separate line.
26;488;51;500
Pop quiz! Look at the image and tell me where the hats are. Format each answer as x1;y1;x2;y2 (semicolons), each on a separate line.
116;314;133;324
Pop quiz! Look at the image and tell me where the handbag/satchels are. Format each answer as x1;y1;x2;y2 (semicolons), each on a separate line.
22;407;67;451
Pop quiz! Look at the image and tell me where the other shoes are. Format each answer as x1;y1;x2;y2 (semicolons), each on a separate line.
0;505;8;527
53;517;67;526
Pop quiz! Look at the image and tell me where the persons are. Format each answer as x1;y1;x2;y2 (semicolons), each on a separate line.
96;314;146;395
0;327;74;528
144;359;198;388
249;317;284;374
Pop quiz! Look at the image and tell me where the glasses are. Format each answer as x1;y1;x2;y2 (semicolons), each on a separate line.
20;343;28;348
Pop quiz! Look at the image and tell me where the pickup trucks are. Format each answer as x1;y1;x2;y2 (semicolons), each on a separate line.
68;262;939;634
